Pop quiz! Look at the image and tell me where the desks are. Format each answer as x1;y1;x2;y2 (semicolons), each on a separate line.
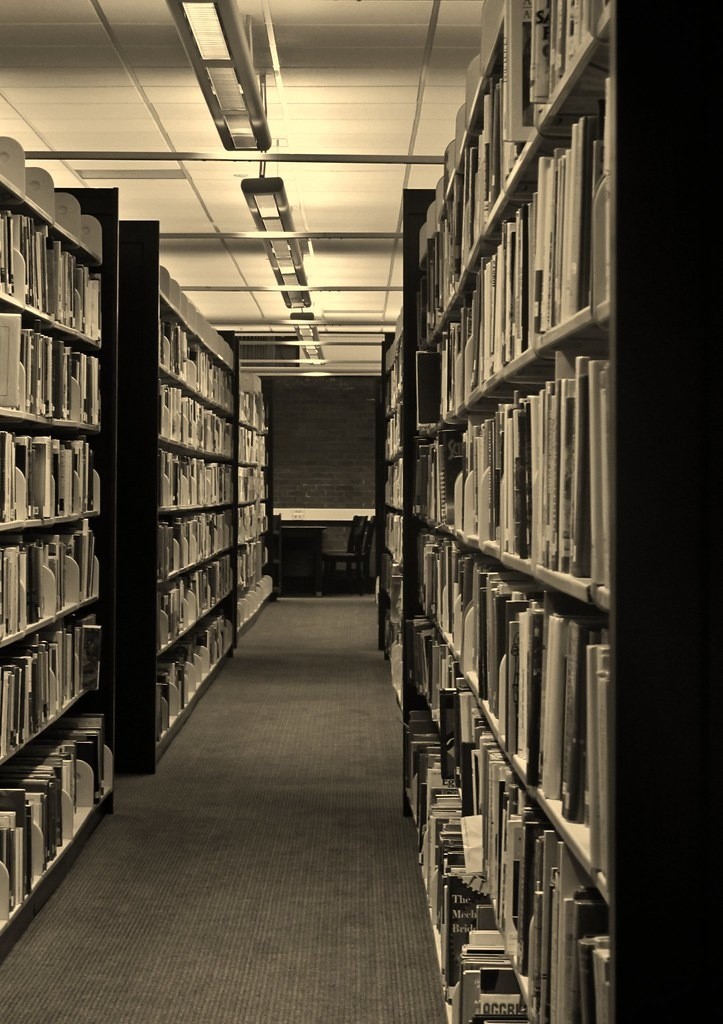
281;525;330;596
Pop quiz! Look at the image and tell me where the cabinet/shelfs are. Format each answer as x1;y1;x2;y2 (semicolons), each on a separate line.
375;0;723;1024
0;134;277;962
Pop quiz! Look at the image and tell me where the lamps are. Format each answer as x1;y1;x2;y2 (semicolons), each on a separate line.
241;161;312;308
165;0;271;152
290;308;325;365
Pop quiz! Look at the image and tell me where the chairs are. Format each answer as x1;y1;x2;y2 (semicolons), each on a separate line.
271;513;284;597
321;515;368;597
328;515;375;592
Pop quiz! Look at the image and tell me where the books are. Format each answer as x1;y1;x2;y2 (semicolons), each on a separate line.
383;0;616;1024
161;299;271;730
1;212;111;913
